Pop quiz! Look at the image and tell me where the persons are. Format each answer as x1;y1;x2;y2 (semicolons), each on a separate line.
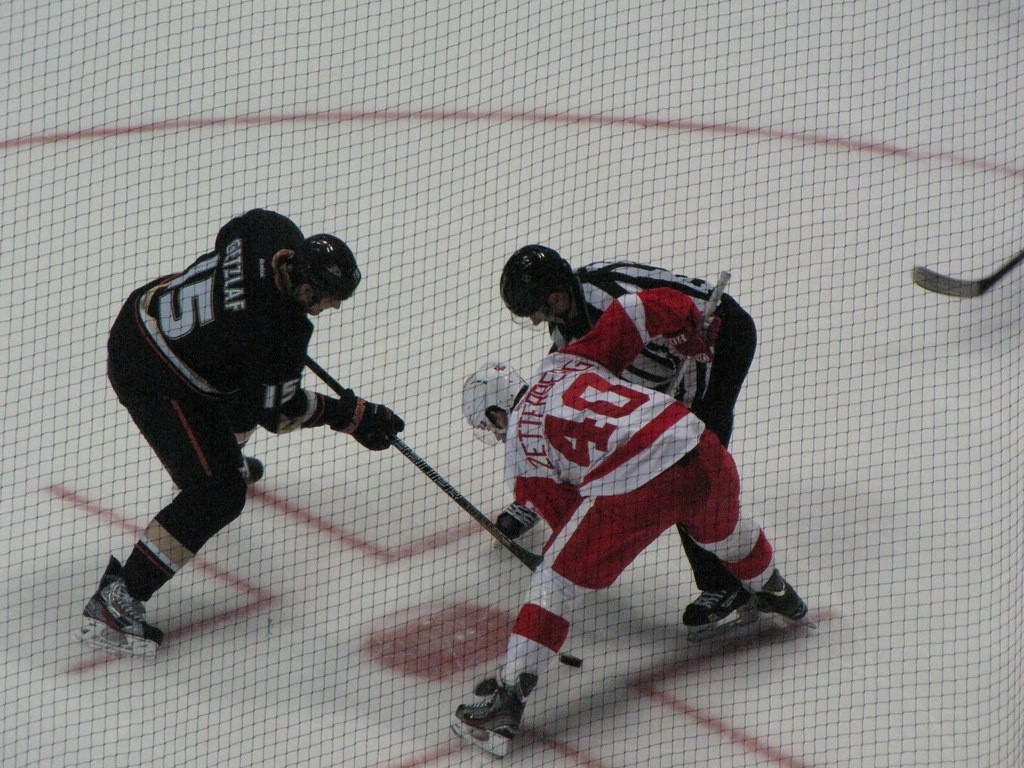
495;246;759;643
72;209;405;659
453;288;816;758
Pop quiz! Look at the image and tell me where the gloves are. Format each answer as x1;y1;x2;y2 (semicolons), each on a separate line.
660;310;721;362
330;389;405;451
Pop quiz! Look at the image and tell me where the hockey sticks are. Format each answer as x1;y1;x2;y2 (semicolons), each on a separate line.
304;354;545;573
666;270;732;398
911;248;1024;299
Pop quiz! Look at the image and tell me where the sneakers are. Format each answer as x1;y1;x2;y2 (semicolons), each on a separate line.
682;575;760;641
74;555;165;656
491;501;539;547
755;568;817;629
237;441;264;484
451;674;539;756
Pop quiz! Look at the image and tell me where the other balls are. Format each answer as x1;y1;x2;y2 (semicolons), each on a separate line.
560;653;583;668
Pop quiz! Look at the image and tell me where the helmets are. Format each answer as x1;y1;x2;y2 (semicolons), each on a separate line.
287;233;362;304
461;362;529;433
500;245;576;317
